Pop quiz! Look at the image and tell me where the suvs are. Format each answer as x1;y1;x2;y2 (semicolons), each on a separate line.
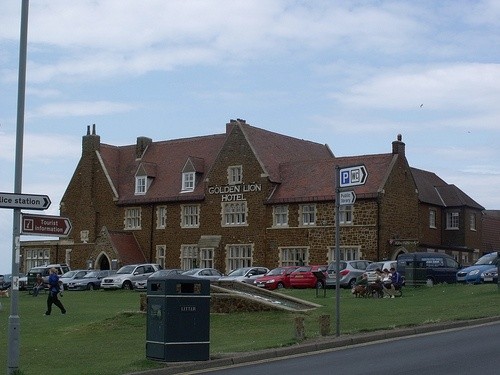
324;260;375;290
99;264;164;291
18;263;72;291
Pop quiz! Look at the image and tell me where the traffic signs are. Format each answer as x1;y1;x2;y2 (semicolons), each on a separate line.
0;192;51;210
339;191;356;205
339;164;368;188
21;213;73;238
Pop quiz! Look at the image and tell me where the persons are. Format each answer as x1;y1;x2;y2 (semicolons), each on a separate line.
44;268;66;316
33;274;44;296
370;267;403;299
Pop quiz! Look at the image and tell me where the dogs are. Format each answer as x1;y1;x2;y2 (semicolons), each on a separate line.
355;286;367;297
366;279;381;298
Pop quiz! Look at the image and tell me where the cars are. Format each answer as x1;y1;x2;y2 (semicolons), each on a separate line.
57;270;92;290
289;265;327;289
135;268;189;292
217;267;270;286
456;252;497;285
66;270;118;292
480;267;500;284
181;268;227;281
253;266;301;290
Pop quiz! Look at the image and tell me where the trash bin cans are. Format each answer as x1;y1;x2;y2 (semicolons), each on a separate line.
405;261;426;288
28;272;42;288
146;270;211;361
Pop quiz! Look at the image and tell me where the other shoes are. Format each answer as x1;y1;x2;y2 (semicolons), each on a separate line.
391;294;395;298
383;295;390;298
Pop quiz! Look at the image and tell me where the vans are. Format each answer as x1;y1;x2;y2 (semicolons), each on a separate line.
0;274;12;291
396;252;465;288
362;261;398;288
